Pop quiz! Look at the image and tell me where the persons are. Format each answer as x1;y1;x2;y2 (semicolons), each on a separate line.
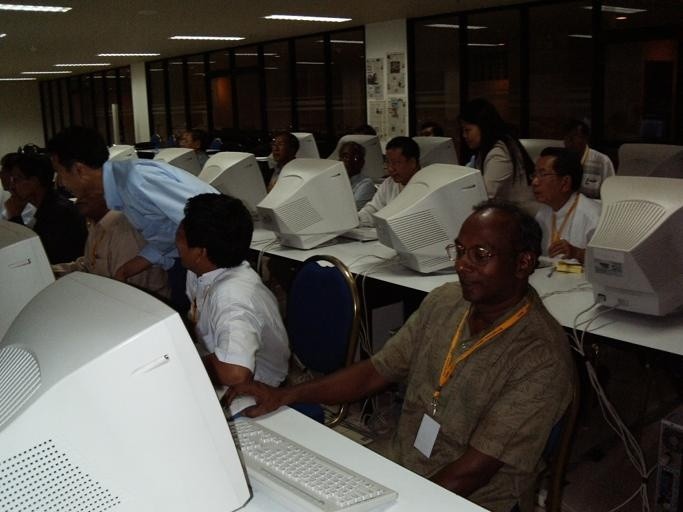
531;145;601;265
456;96;536;210
219;194;580;512
562;118;616;200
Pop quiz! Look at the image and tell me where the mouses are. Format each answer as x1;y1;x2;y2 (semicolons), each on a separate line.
221;395;255;422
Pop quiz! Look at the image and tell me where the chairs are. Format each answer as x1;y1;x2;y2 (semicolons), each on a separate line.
528;360;581;511
280;252;363;429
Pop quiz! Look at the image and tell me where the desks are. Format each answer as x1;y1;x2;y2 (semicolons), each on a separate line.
215;378;492;511
241;217;405;347
350;255;680;427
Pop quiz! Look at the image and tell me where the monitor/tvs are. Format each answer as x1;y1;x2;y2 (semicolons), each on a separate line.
255;157;360;250
410;136;459;169
196;152;264;216
327;135;385;179
371;164;488;274
615;142;683;179
584;176;683;319
107;144;138;163
255;157;271;191
289;132;320;160
0;220;53;340
0;270;253;512
518;138;566;167
154;148;200;178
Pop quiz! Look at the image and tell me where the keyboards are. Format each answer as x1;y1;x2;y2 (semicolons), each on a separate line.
342;228;377;243
226;419;398;512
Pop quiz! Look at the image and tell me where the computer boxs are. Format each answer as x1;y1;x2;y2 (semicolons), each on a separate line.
655;405;683;512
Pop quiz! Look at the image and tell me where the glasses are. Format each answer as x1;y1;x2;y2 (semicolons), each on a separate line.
446;244;503;264
